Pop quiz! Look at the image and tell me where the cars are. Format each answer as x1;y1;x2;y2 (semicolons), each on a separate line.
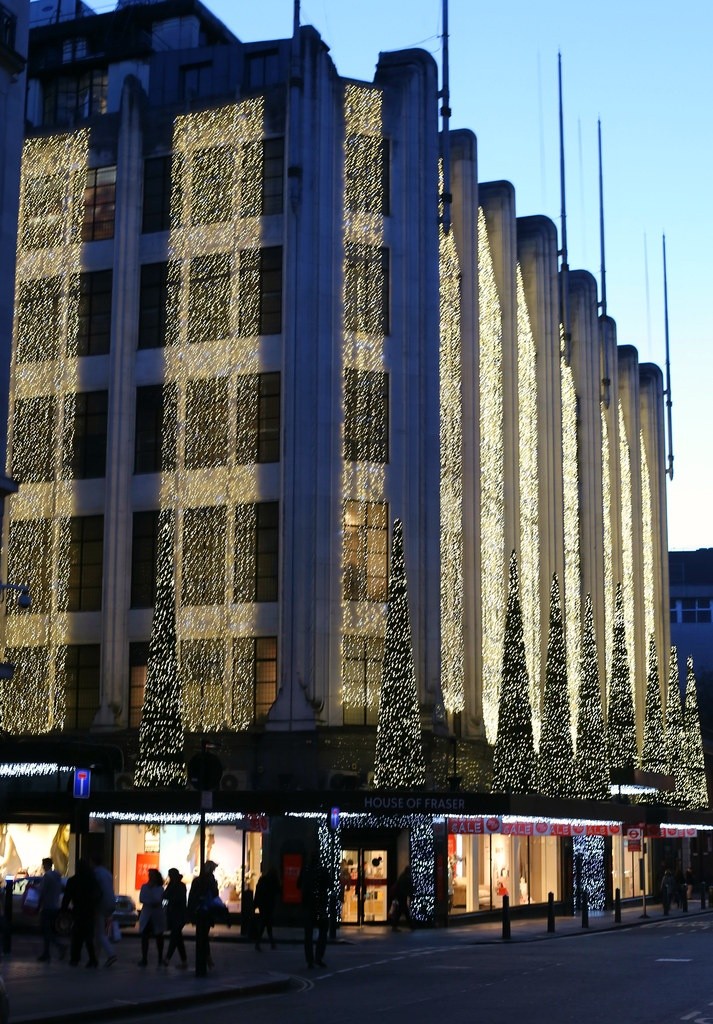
37;892;139;933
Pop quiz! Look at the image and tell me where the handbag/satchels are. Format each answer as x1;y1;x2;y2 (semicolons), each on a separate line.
19;878;41;916
24;887;40;908
107;920;122;944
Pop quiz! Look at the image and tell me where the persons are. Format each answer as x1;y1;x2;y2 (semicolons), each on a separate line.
338;860;352;906
186;860;227;973
50;823;71;879
0;822;26;890
296;846;332;969
387;865;418;932
248;868;282;952
659;860;695;916
20;851;119;969
137;868;188;969
187;822;216;878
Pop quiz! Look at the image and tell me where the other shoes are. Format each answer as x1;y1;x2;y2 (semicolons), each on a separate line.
270;945;277;949
105;955;117;967
315;958;326;968
208;957;215;970
138;960;147;967
307;963;316;970
59;950;65;961
254;945;262;952
176;963;187;969
38;954;51;961
162;960;168;967
85;958;99;968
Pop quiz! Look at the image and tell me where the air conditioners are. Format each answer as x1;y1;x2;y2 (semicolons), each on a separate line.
114;772;133;789
322;770;357;791
220;771;247;790
